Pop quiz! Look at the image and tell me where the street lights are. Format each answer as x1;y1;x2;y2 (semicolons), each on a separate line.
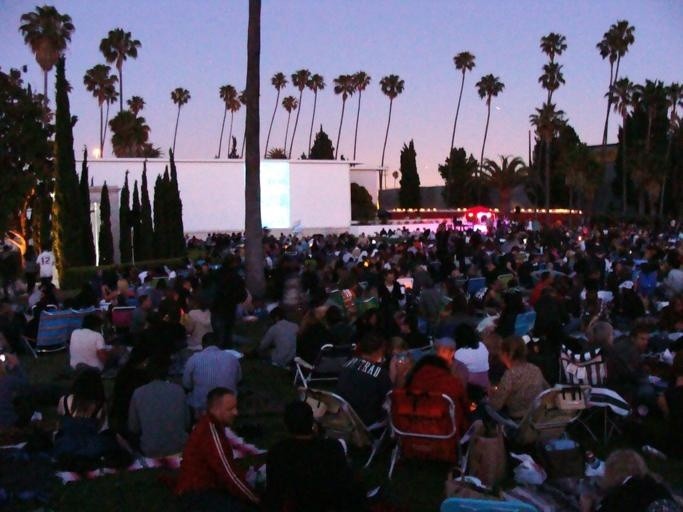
555;387;586;410
302;395;328;417
446;419;599;511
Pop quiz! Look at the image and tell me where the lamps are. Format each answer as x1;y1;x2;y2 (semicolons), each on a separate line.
21;303;92;360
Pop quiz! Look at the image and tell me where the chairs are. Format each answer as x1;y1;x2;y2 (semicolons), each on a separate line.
21;303;92;360
111;274;608;482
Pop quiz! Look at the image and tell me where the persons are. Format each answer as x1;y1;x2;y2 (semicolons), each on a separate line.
0;221;683;512
